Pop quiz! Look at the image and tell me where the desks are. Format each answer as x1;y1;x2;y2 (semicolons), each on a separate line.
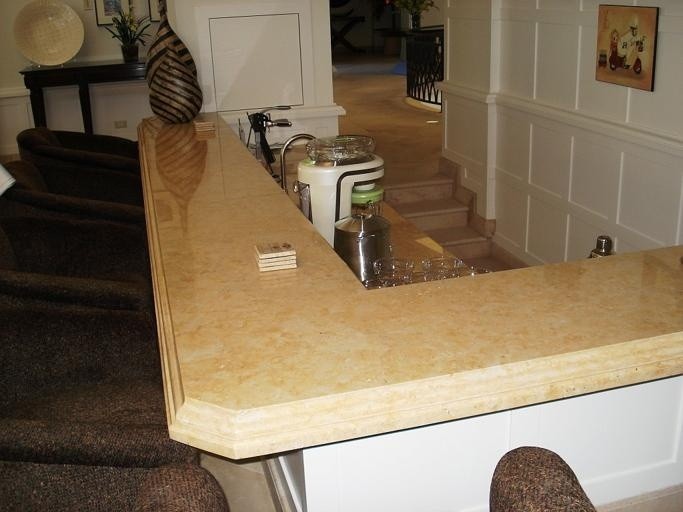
20;55;155;138
331;15;365;56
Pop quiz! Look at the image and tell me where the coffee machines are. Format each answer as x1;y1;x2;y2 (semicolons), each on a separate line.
296;135;386;250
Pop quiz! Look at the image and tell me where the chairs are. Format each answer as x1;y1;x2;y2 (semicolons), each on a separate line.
0;271;232;512
14;126;139;169
0;153;150;278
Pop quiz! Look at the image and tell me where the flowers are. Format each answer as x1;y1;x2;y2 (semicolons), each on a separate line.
104;5;152;47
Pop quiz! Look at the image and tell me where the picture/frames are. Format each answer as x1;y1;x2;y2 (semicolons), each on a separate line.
149;1;161;21
94;1;132;26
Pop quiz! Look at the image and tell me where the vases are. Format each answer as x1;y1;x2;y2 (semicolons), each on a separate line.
121;44;139;62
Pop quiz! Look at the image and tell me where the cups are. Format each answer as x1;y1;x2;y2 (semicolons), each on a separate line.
362;254;494;292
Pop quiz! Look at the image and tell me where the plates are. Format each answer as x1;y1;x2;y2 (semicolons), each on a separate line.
13;0;85;66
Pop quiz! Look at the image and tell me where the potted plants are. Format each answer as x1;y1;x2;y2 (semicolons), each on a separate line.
395;1;432;30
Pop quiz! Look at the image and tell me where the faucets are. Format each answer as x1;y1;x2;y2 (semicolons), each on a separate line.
279;133;316;196
